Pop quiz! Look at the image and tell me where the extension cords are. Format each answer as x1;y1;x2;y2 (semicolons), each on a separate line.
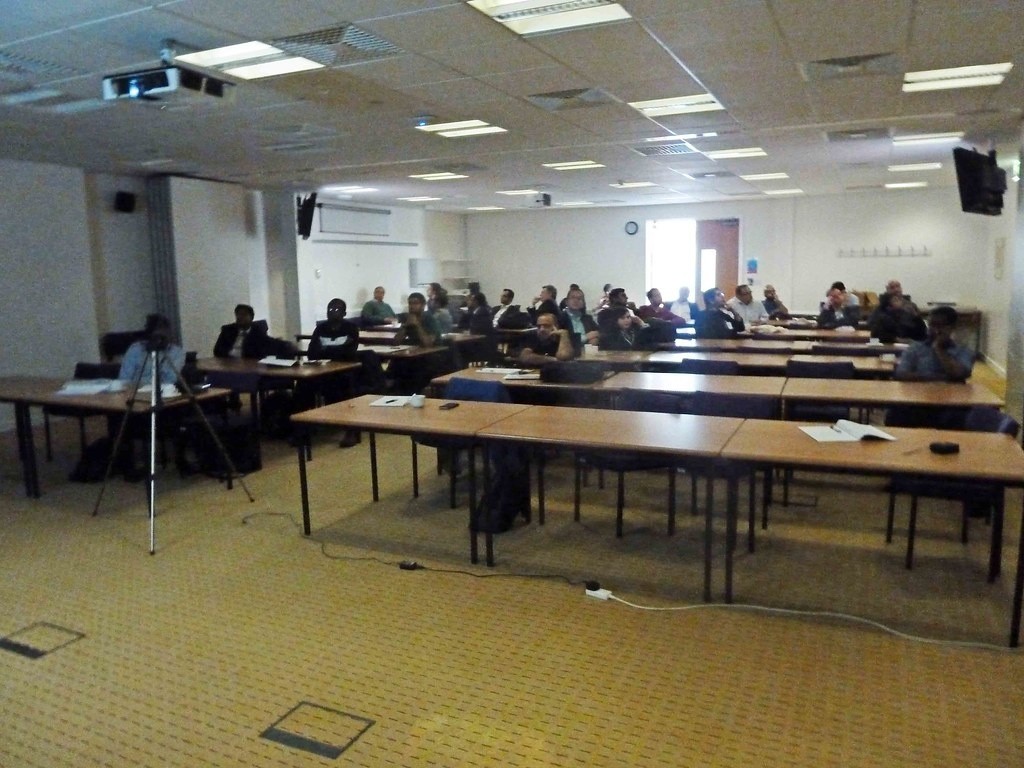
585;587;612;601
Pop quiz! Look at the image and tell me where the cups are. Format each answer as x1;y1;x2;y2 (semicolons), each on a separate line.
870;338;879;345
883;353;895;363
110;380;122;390
411;395;425;407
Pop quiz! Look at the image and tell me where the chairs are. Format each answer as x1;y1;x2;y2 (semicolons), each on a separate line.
571;384;685;536
407;375;509;504
39;359;122;460
685;397;772;532
779;359;855;421
882;403;1021;570
537;358;606;488
101;329;144;357
680;358;742;413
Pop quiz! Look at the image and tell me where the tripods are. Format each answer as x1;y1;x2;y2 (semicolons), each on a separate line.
92;333;256;556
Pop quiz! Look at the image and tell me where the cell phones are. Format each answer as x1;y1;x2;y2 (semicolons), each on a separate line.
438;402;460;410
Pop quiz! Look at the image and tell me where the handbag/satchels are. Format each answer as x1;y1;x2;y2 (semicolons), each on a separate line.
468;470;529;533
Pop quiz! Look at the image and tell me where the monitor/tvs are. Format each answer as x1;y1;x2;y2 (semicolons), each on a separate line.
298;192;317;241
953;147;1006;217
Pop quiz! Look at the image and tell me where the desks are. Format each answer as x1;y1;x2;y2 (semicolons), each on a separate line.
1;313;1024;648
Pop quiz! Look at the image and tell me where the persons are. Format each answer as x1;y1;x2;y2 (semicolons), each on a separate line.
117;314;188;481
290;281;976;491
212;303;270;358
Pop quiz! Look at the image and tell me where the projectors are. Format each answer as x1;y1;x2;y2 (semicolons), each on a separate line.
101;64;238;110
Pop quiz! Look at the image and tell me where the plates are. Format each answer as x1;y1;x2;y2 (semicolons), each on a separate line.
866;344;883;346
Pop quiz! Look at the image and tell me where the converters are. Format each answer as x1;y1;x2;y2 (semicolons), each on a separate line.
399;560;417;570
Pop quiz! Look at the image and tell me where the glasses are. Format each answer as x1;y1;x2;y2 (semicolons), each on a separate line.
328;306;344;312
765;290;776;294
708;292;725;297
536;324;554;329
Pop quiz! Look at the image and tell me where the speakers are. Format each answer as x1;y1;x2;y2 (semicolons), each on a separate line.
114;191;137;212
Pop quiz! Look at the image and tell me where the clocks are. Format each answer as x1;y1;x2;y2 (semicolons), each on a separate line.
625;221;638;234
